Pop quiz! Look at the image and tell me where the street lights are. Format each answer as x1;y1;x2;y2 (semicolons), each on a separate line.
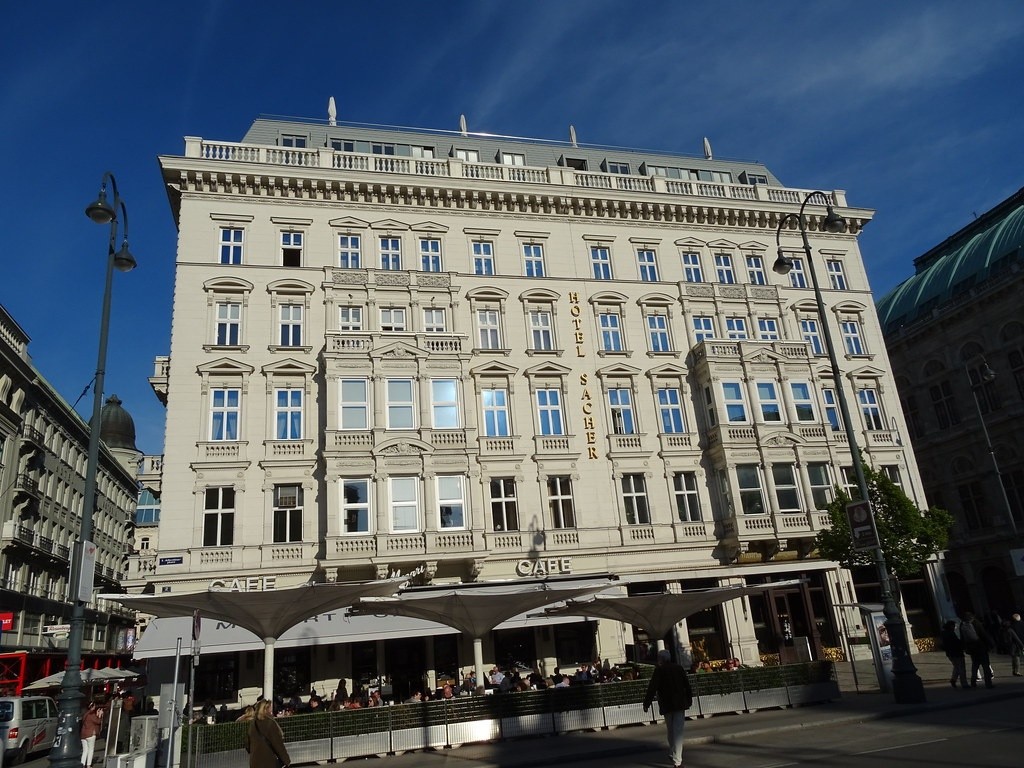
775;191;932;719
45;171;137;768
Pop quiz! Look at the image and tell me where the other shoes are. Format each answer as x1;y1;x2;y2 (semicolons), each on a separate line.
950;679;957;689
977;678;981;681
963;685;971;689
991;675;994;678
1015;672;1022;676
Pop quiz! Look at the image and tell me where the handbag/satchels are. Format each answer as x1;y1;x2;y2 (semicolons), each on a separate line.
670;676;689;703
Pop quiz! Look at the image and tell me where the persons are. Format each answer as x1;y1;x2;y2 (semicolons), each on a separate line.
984;608;1001;644
962;612;995;687
690;658;739;674
106;692;136;710
276;692;283;705
405;671;475;704
942;620;970;692
290;693;302;705
248;699;291;768
80;701;103;768
237;707;254;721
491;656;616;692
143;702;158;714
1001;613;1024;677
335;678;349;698
643;650;693;768
309;687;383;712
182;700;227;718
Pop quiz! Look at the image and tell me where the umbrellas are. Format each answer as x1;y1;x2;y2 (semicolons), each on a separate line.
95;576;409;711
21;669;139;699
347;580;628;695
528;578;812;650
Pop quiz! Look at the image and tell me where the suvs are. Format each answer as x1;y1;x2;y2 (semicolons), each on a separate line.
0;693;62;766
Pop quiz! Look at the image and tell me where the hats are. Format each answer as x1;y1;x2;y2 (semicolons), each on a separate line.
657;650;670;660
